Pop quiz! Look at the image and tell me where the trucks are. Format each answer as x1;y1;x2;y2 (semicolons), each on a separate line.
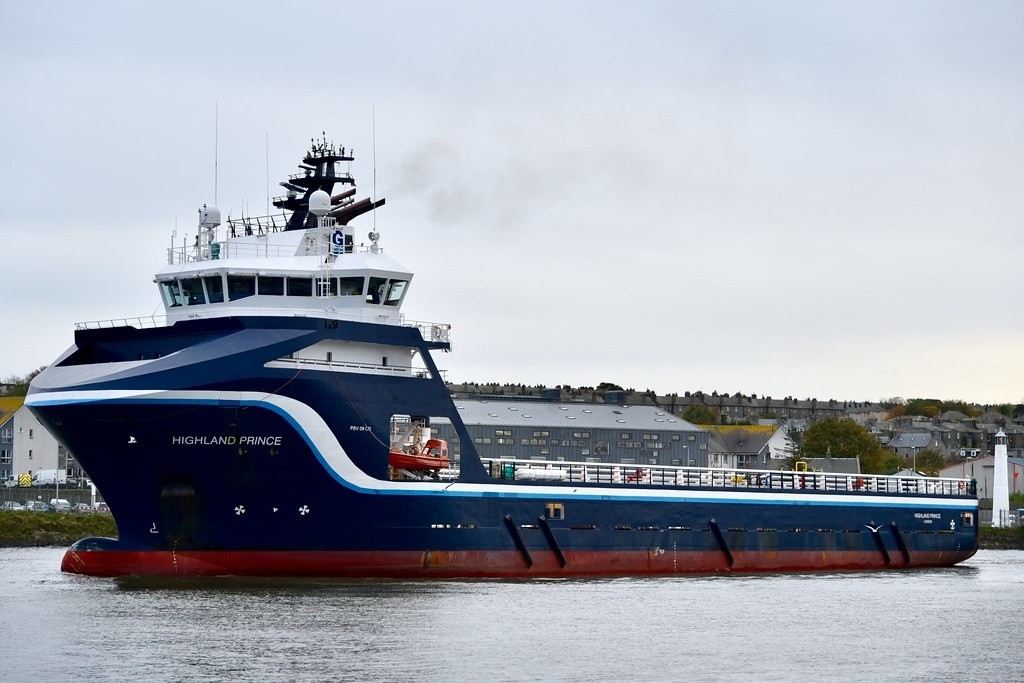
31;469;66;489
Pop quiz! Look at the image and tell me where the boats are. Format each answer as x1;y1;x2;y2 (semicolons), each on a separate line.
24;103;979;580
389;439;450;468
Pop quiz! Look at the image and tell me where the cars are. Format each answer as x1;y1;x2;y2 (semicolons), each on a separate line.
3;502;24;511
73;503;90;513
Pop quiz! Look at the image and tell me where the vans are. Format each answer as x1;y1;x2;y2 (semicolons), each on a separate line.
50;498;71;513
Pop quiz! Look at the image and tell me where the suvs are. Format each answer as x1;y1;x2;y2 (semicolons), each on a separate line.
24;501;46;511
